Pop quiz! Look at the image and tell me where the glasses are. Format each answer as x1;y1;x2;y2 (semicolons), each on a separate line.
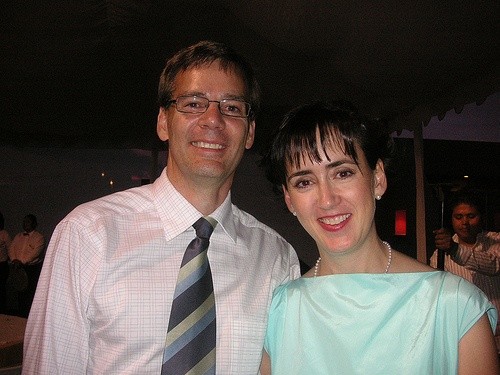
166;95;252;118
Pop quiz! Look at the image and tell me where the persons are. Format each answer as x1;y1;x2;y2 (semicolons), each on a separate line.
254;95;500;375
21;41;301;374
429;191;500;354
0;212;46;318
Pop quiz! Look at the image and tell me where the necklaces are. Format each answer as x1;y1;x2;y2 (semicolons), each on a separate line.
313;241;392;278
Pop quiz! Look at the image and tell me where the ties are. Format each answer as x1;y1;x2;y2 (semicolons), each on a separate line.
162;216;217;375
